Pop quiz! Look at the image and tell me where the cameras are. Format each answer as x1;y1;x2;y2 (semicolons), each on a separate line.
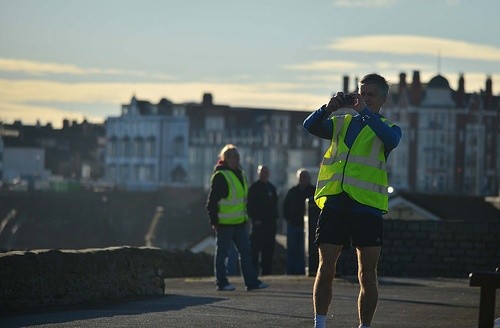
340;94;357;106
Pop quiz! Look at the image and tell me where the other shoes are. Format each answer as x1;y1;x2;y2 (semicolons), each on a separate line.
217;284;235;291
246;282;268;292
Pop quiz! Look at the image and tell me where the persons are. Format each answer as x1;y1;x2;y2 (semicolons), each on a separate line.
302;73;402;328
246;163;277;275
283;167;320;277
206;144;269;291
